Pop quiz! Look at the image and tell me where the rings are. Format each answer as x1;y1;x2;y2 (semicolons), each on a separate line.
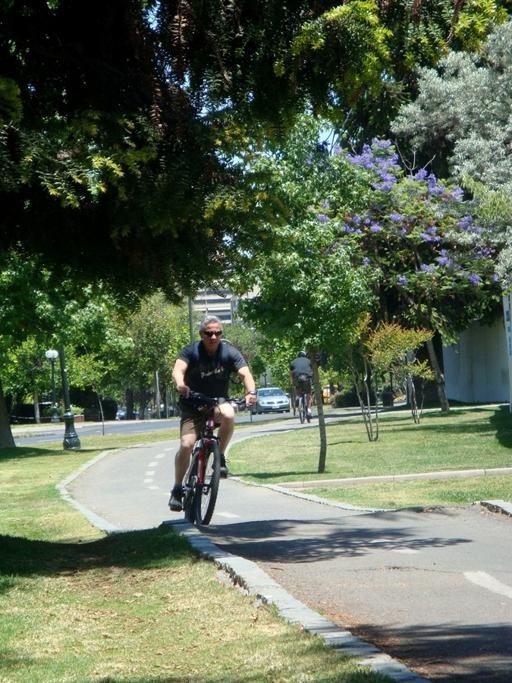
184;390;186;392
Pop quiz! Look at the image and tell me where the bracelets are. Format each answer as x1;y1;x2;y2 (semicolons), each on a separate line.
247;390;257;394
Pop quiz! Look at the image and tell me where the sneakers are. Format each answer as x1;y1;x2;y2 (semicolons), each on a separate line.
295;397;299;408
306;413;313;419
212;455;228;474
169;487;183;508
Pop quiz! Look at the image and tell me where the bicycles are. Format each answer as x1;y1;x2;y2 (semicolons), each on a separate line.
297;374;311;423
175;385;247;532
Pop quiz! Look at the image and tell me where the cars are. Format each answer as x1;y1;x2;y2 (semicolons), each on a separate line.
116;404;178;419
251;386;290;414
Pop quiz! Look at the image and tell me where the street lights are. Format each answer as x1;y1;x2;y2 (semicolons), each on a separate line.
44;349;60;422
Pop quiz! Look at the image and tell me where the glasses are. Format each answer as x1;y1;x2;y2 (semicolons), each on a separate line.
204;330;222;336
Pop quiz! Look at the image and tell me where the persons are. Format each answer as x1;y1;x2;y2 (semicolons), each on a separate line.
290;350;315;418
167;315;258;510
